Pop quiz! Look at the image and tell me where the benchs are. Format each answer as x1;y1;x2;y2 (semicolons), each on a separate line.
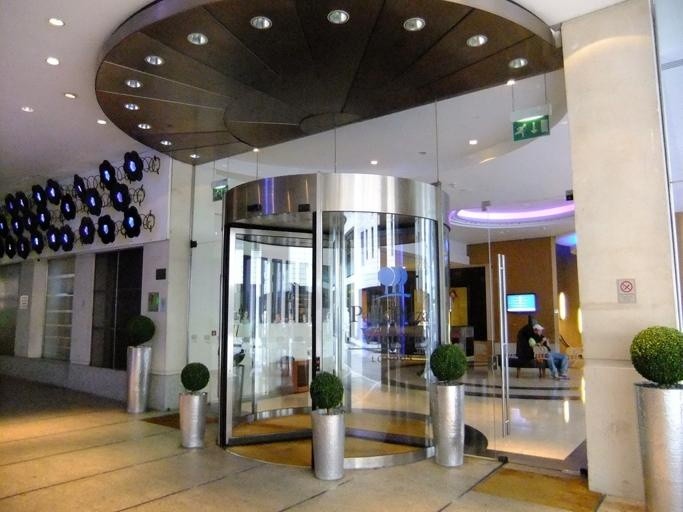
497;353;548;377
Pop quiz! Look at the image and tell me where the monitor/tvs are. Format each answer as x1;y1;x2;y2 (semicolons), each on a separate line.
507;293;537;313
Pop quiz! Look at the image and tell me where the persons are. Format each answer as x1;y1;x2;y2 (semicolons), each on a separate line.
528;324;569;380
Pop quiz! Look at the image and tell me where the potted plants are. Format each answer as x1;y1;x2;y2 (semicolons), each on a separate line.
630;326;683;512
125;316;155;413
309;372;345;480
179;363;210;448
428;344;467;467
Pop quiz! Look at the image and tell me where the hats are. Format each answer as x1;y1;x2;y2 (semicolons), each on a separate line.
532;323;545;330
